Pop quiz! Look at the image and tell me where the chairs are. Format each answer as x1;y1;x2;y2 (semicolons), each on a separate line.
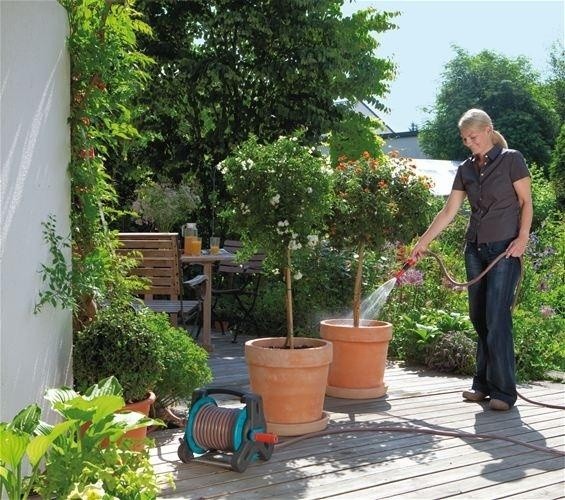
196;240;268;343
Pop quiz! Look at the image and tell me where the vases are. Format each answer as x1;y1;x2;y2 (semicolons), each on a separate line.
319;318;393;389
244;337;333;424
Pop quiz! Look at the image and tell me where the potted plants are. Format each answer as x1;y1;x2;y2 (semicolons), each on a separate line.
73;308;181;464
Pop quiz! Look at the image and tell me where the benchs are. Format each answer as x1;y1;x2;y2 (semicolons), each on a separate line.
72;232;209;339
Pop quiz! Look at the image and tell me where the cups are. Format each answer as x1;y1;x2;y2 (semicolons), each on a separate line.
209;237;221;256
191;237;202;256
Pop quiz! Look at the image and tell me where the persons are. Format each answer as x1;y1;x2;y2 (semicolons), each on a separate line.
408;108;533;410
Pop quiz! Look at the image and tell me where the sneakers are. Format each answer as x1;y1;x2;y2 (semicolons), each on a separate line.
489;399;510;411
463;389;483;402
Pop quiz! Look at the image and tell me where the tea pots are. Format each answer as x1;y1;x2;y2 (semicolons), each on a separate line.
182;222;198;255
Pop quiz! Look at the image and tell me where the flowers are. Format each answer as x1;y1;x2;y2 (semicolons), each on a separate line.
317;150;444;324
214;125;339;347
